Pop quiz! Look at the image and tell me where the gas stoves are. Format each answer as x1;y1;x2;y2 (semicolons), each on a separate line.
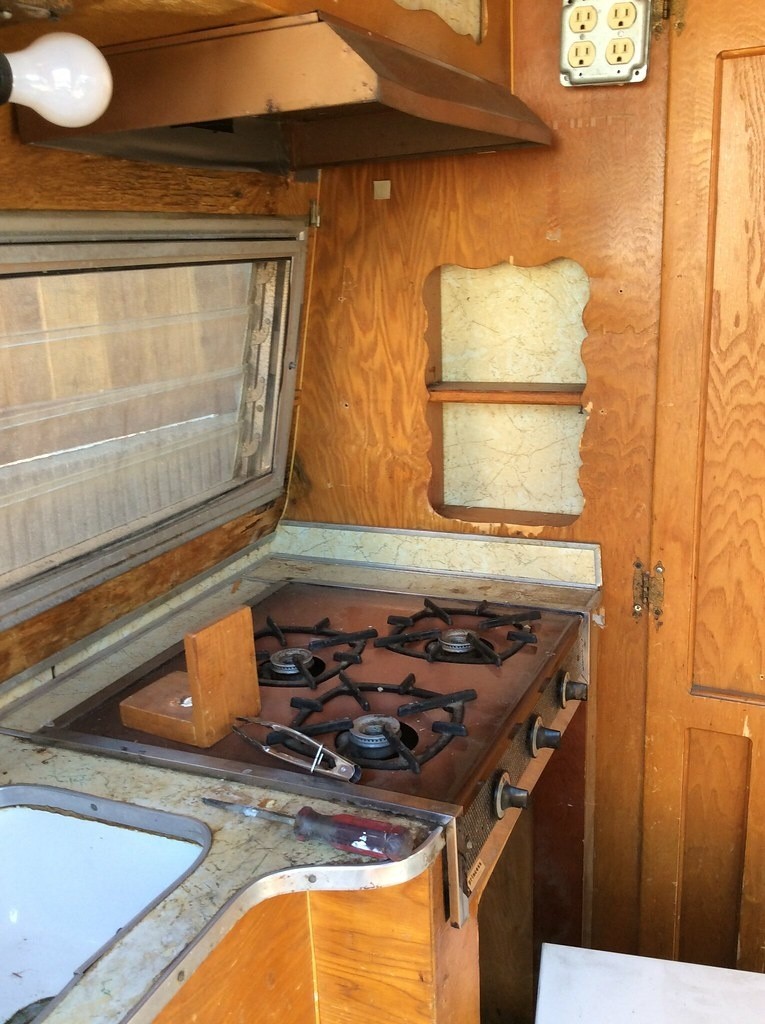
70;578;590;896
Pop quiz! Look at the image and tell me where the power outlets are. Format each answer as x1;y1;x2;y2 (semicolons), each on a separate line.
555;0;653;89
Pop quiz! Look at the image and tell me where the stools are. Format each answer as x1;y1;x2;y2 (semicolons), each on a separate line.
532;940;765;1024
